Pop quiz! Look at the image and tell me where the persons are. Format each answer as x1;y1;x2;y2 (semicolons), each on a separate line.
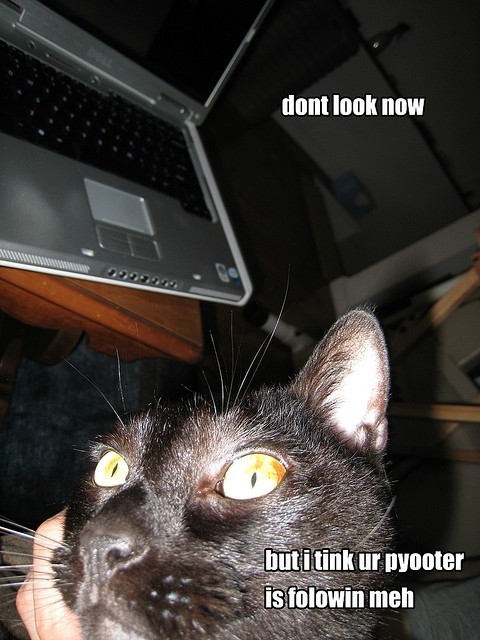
15;503;82;640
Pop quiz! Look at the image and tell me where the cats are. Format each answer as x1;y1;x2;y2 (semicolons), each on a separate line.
1;305;403;639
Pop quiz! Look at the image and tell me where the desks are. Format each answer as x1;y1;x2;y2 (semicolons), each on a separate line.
0;257;204;365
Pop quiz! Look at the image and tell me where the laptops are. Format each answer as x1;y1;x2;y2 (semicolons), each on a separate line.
0;0;273;310
